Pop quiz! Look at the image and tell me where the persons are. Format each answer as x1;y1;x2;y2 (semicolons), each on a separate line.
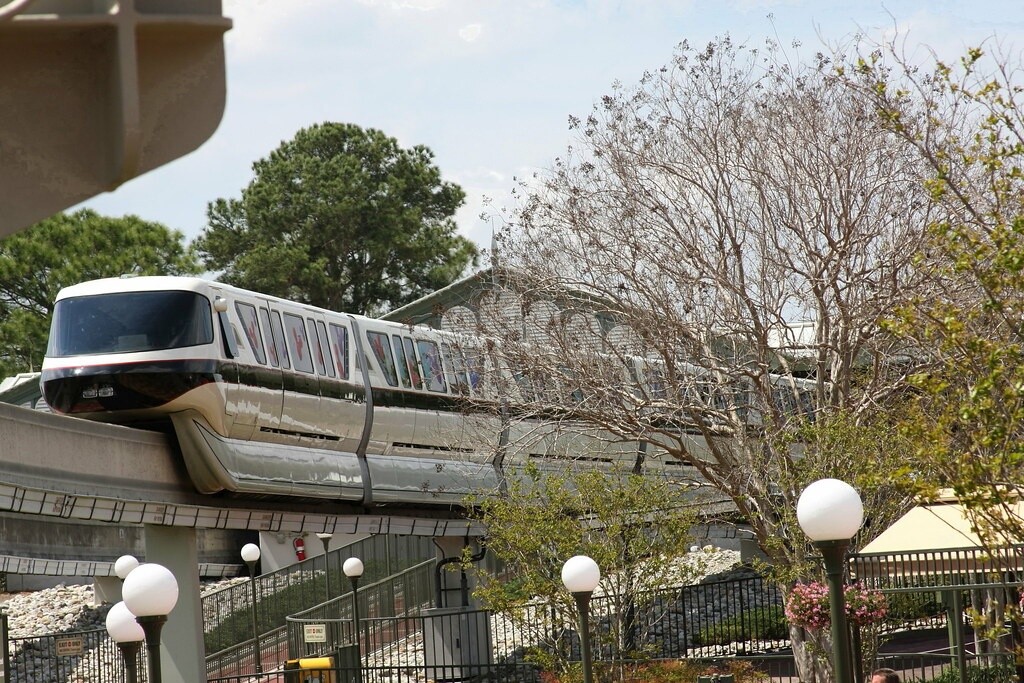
872;668;902;683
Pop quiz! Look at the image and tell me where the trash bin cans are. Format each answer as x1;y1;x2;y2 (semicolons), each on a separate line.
284;655;338;683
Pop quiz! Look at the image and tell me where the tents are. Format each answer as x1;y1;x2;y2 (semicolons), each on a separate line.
847;482;1024;642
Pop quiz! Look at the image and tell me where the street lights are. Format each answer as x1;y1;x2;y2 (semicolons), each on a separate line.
343;557;365;647
240;542;264;676
794;477;865;681
561;554;601;683
105;600;145;683
317;533;332;602
123;562;179;683
115;555;138;583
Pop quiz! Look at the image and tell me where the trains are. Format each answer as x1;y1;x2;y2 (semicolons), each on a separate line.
38;274;831;477
